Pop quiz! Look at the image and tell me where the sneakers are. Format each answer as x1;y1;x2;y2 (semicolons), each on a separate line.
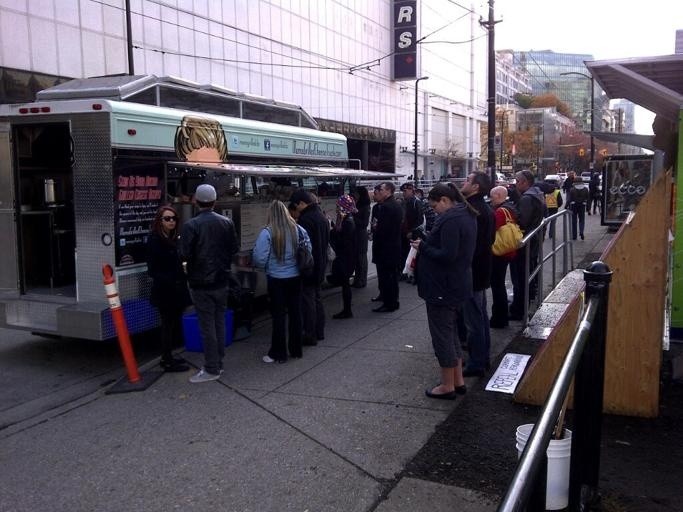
333;310;352;319
426;384;466;399
463;365;490;377
159;358;189;372
263;354;302;364
304;336;324;346
189;366;224;383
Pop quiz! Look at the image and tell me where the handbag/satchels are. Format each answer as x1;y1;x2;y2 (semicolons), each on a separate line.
297;241;315;270
492;222;523;257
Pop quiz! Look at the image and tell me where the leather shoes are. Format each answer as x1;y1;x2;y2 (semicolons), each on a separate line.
371;296;383;302
372;303;395;313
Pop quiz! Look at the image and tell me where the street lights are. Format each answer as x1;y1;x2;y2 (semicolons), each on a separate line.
413;76;430;187
560;72;594;166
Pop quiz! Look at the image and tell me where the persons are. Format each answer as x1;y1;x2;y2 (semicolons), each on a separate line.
147;207;194;371
253;170;600;401
178;184;238;383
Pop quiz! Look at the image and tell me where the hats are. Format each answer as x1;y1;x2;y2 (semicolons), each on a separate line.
335;195;358;215
196;184;216;202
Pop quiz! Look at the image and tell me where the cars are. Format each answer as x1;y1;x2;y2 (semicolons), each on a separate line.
495;171;591;185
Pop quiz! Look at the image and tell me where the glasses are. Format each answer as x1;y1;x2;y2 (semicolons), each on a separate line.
161;215;177;221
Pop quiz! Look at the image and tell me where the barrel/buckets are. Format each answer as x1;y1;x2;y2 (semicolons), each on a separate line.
515;424;572;512
